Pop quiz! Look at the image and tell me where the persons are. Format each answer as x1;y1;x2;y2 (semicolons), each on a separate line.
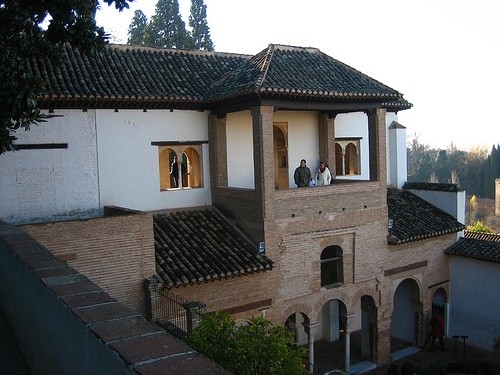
283;316;300;350
314;160;332;186
429;309;451;352
294;158;311;188
170;155;185;188
